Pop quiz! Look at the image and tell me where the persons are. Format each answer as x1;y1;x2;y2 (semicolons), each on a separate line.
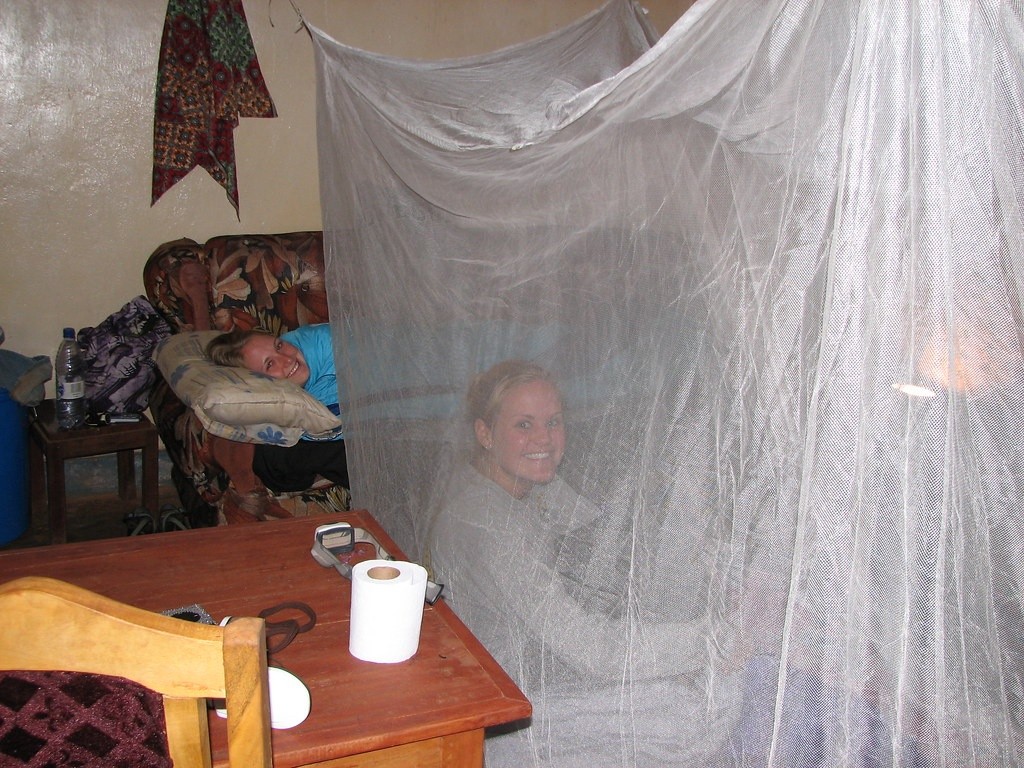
417;363;757;767
205;323;645;443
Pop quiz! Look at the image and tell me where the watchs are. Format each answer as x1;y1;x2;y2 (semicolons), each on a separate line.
311;522;445;605
84;408;111;427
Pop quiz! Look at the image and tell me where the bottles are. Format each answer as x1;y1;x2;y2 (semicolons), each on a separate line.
55;328;85;428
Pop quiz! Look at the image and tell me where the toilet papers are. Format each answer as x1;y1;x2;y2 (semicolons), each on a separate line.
348;559;428;664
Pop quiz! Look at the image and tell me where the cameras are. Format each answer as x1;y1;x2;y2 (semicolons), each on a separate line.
85;406;111;426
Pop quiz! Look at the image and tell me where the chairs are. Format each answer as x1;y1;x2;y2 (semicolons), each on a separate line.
1;575;273;767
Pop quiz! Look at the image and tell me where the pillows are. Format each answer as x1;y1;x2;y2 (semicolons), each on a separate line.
157;330;341;434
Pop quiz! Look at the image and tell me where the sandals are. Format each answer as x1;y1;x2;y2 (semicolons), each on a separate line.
125;504;191;536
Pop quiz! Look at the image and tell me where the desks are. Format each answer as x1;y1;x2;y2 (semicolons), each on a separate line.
23;398;159;548
1;510;532;768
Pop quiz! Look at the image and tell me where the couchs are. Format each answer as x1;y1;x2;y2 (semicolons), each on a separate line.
148;231;614;529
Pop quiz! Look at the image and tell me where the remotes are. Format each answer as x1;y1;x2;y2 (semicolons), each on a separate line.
109;413;143;423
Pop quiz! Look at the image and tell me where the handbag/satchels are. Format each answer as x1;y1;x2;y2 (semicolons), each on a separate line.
77;296;175;425
